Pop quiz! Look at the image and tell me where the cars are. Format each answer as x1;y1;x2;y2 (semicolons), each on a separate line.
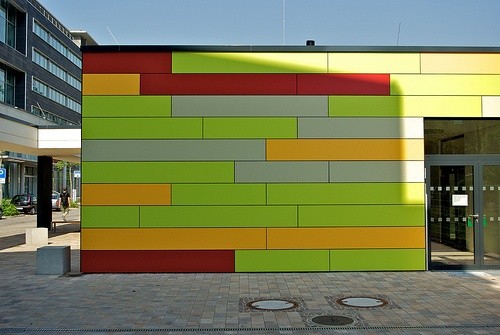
51;194;61;210
10;194;37;214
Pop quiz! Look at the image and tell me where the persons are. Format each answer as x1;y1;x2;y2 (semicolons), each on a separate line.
59;188;71;222
